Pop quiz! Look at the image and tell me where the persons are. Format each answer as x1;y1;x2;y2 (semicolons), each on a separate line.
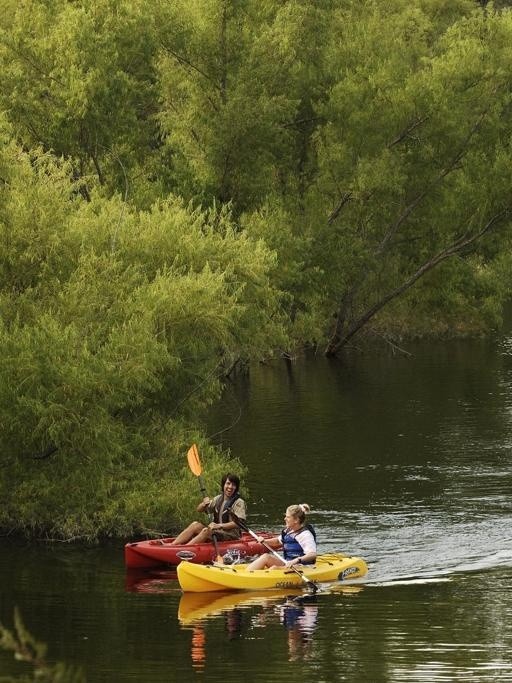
224;608;243;643
245;503;318;572
279;591;319;662
159;473;247;546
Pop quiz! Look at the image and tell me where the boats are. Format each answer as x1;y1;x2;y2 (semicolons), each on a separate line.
125;531;367;592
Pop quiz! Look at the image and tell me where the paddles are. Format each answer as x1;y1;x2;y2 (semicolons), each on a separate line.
227;508;320;592
187;442;223;565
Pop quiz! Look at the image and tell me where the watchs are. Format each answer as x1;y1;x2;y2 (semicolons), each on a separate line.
296;556;302;563
